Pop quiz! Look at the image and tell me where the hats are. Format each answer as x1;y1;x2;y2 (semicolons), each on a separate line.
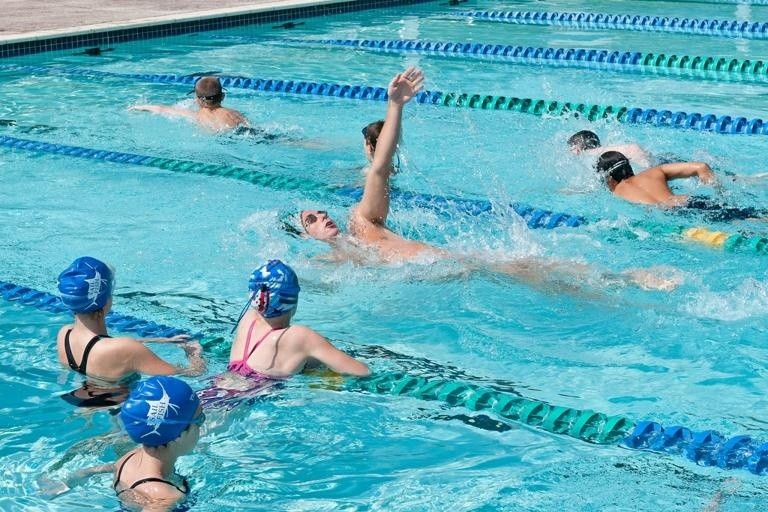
249;259;299;317
122;375;200;446
57;256;115;311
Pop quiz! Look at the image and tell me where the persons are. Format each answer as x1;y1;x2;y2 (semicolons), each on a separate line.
106;375;205;512
123;73;348;152
29;260;370;504
563;129;768;182
328;118;397;191
558;150;767;227
47;255;206;412
275;64;692;316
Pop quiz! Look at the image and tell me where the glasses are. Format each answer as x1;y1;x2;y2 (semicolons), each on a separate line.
193;410;206;427
304;209;327;228
